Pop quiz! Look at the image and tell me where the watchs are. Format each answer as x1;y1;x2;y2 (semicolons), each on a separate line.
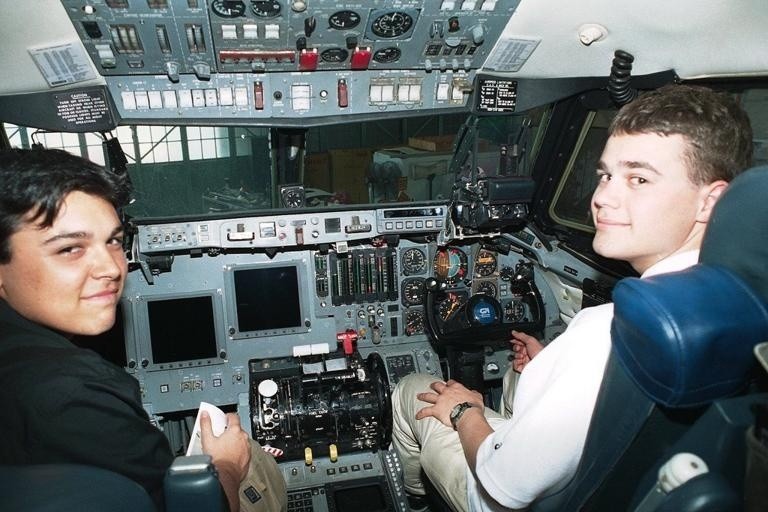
450;402;482;431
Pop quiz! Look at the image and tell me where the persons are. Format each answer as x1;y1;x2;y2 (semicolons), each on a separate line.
0;150;287;512
390;83;752;512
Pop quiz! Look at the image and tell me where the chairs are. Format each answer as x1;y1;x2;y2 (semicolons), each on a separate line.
1;451;232;512
406;161;768;511
